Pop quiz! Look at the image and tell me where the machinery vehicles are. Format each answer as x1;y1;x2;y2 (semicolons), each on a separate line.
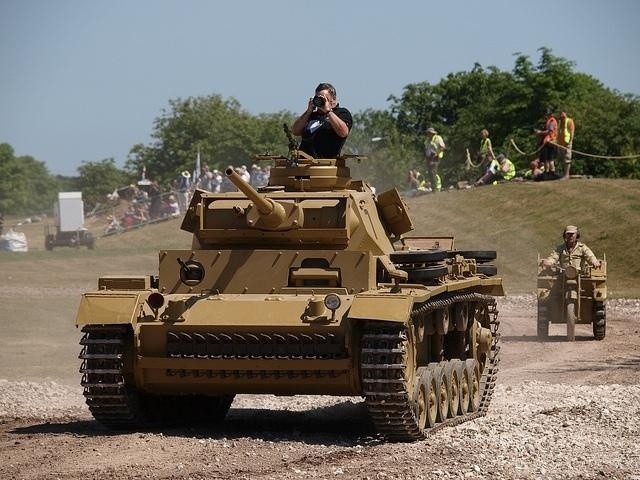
71;118;507;442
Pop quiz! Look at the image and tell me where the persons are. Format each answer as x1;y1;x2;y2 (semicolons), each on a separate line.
539;223;602;276
525;108;574;179
106;165;271;234
405;128;446;196
474;130;515;187
291;83;352;160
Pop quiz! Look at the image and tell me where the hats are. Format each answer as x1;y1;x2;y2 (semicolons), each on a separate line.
564;225;578;235
497;154;504;159
181;170;191;178
424;127;438;134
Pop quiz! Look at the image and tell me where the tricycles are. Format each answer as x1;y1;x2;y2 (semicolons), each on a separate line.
535;250;608;343
